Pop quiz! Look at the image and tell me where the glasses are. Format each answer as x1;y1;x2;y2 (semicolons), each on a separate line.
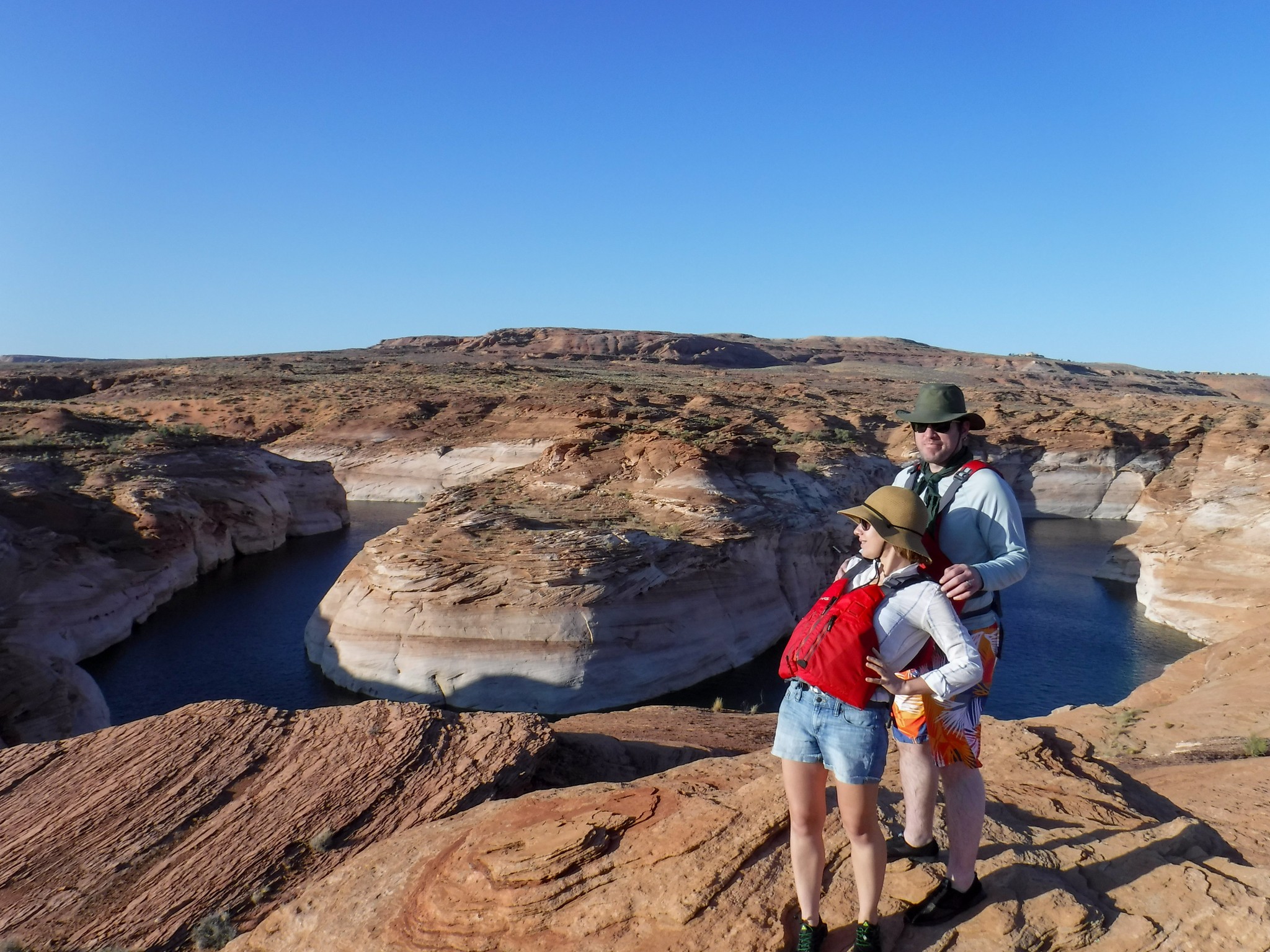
859;517;871;531
911;420;961;433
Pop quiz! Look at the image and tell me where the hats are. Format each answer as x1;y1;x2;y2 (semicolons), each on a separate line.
895;383;986;431
836;486;932;565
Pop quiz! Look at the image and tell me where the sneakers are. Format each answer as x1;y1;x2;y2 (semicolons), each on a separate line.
795;913;828;952
852;919;882;952
885;830;941;863
904;871;983;927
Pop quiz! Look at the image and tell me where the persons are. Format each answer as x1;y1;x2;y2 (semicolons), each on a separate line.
885;381;1030;928
771;486;983;951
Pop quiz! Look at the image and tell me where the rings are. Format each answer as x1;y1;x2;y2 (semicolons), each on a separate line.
966;580;972;587
